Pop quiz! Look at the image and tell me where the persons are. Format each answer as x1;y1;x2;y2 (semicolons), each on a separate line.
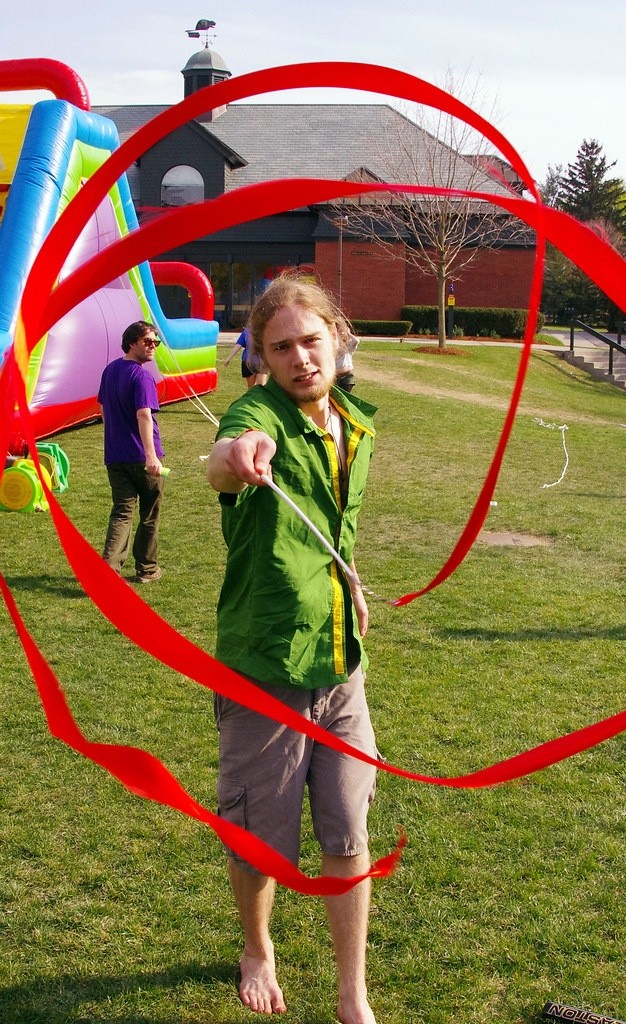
335;326;360;394
96;321;164;584
207;268;386;1024
224;331;266;390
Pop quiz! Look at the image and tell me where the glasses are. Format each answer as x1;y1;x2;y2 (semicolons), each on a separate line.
142;338;161;347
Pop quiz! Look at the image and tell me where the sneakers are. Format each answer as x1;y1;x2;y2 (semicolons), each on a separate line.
136;567;161;583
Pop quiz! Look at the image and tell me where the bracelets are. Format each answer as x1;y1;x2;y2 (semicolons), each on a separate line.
234;428;262;442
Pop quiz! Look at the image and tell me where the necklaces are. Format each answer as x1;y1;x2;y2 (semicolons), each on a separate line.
315;402;345;484
